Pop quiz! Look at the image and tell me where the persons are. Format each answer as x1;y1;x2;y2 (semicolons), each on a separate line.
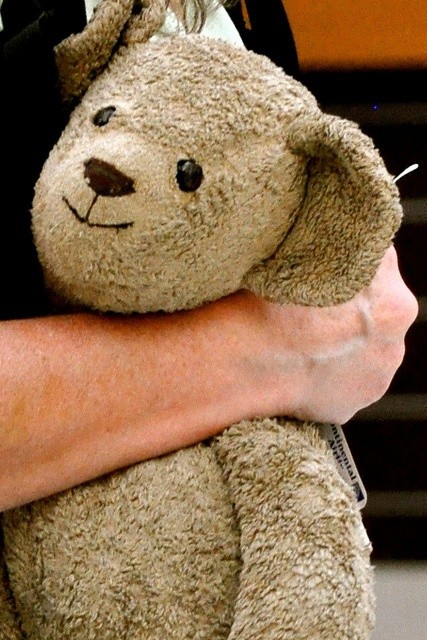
0;1;419;513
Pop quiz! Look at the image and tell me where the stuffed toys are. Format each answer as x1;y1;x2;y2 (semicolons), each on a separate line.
1;0;403;638
